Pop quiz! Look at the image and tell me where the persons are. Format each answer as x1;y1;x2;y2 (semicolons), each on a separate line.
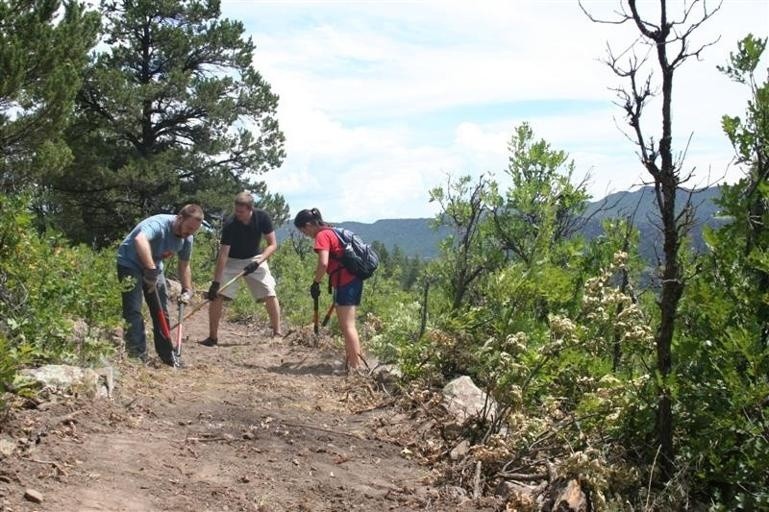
197;192;283;346
117;204;204;367
293;207;363;374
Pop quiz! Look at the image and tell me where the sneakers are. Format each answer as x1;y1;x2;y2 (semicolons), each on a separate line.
200;337;217;346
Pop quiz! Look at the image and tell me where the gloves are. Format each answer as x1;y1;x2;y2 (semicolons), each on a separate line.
142;268;161;294
243;261;259;276
177;288;192;304
310;280;321;298
208;280;221;301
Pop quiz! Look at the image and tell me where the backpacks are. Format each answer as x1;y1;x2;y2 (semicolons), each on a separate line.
333;227;380;280
